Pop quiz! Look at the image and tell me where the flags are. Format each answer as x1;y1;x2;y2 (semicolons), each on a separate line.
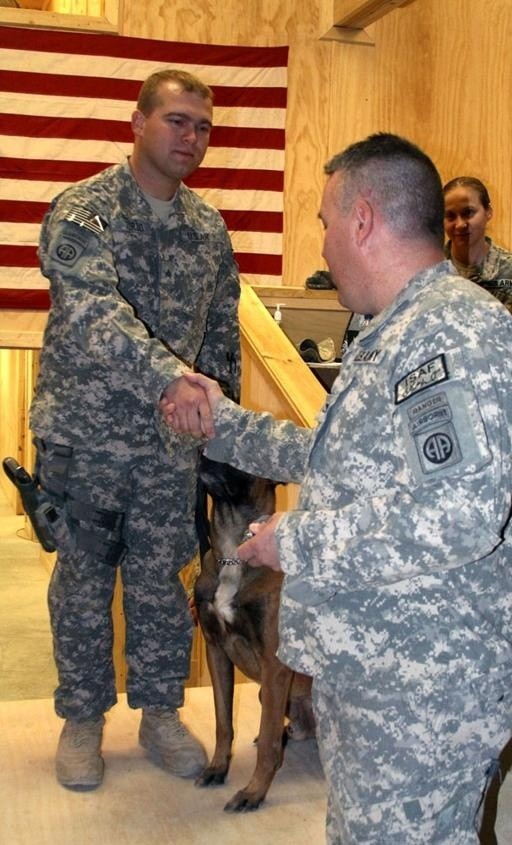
0;29;289;312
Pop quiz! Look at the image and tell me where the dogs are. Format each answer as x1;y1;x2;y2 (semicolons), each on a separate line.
193;452;316;813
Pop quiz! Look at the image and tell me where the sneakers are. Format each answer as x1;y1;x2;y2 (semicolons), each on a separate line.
138;707;208;778
55;714;106;792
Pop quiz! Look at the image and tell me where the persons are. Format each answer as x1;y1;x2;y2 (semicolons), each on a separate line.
155;132;511;845
24;68;243;789
444;176;512;315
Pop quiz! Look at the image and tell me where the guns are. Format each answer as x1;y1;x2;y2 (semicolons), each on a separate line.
2;456;57;552
